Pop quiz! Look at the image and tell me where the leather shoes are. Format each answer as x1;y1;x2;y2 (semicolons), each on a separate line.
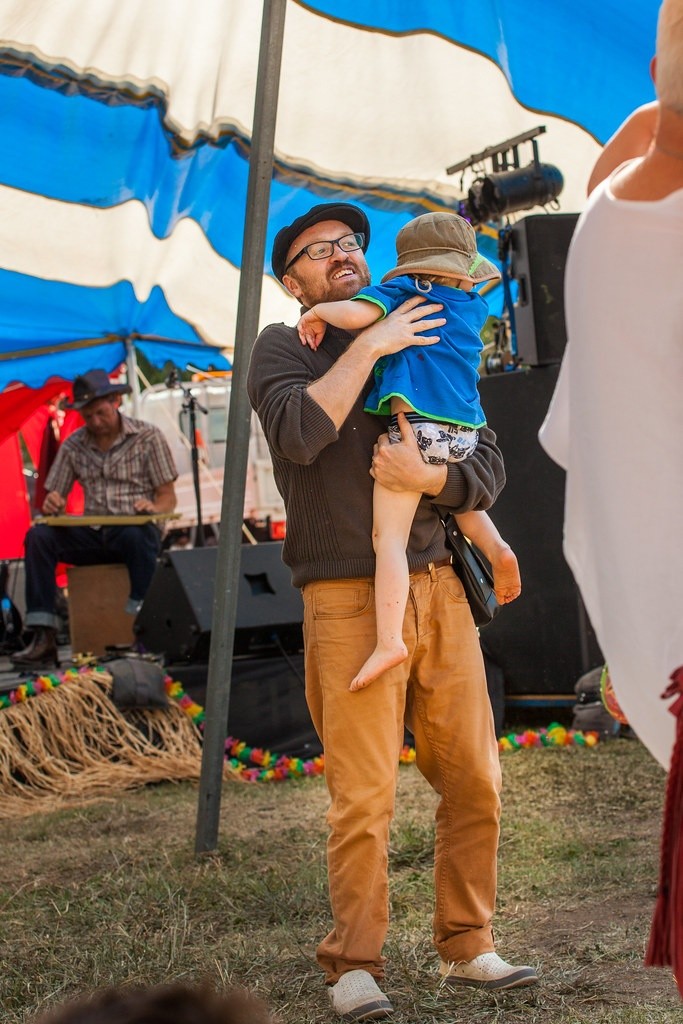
11;630;59;665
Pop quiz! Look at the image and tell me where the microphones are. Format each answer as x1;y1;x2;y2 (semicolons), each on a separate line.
165;368;177;388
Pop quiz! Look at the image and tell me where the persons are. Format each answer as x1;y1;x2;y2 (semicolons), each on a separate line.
243;198;542;1024
10;364;180;668
294;207;523;693
20;974;274;1024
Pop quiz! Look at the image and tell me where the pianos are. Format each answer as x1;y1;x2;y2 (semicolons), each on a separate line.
34;510;183;527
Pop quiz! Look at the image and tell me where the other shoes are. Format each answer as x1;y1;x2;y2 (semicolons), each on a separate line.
439;952;538;991
328;969;394;1023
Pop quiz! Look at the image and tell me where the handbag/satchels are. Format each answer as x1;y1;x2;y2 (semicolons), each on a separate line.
445;517;504;623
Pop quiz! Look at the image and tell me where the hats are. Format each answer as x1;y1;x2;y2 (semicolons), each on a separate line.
272;207;369;280
380;211;503;295
58;370;127;410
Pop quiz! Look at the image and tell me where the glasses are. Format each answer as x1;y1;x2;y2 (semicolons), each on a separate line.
282;232;365;276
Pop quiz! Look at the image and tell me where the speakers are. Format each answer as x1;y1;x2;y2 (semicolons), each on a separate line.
133;541;304;665
508;212;582;367
